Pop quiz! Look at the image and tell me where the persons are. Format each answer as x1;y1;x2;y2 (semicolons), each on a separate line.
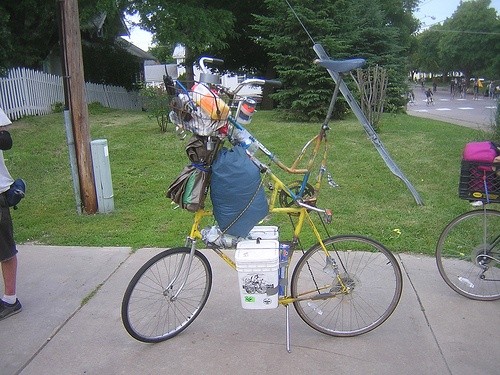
408;76;500;106
0;108;23;321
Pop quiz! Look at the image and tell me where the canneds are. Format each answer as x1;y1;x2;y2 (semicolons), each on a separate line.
280;244;290;263
236;99;256;125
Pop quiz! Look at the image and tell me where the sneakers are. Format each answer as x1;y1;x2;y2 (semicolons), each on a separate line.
0;298;23;321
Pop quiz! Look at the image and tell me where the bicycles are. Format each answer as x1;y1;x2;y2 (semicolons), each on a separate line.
436;145;500;301
121;57;403;353
409;81;495;108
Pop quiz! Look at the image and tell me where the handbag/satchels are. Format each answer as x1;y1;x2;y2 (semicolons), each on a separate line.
6;178;26;207
463;142;500;189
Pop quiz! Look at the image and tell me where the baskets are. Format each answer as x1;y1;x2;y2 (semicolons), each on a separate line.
459;160;500;202
165;80;245;143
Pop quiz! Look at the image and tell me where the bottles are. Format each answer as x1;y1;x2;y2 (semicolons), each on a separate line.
237;99;256;124
192;84;229;120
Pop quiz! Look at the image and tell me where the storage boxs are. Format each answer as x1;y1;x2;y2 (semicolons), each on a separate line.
458;159;500;203
234;226;279;310
